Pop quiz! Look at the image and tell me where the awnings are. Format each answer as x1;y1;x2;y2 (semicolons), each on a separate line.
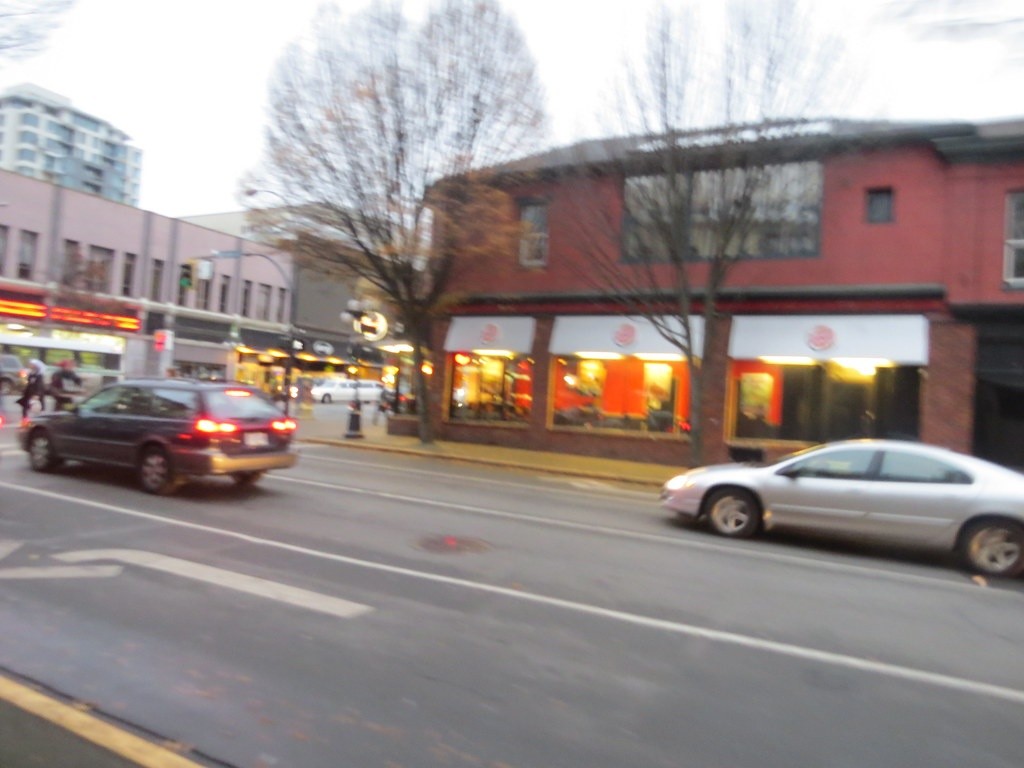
728;313;929;366
549;314;704;358
443;317;536;352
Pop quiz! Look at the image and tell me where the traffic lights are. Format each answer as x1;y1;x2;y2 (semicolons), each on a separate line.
178;259;199;292
153;329;173;351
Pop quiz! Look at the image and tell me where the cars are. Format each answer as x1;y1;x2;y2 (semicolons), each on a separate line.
656;438;1023;583
16;375;301;499
0;354;30;397
277;377;398;414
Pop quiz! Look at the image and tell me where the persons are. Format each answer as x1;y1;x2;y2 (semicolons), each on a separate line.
166;367;176;376
50;359;81;412
21;358;47;425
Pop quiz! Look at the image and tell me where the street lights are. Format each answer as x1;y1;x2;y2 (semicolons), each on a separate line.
242;185;302;416
